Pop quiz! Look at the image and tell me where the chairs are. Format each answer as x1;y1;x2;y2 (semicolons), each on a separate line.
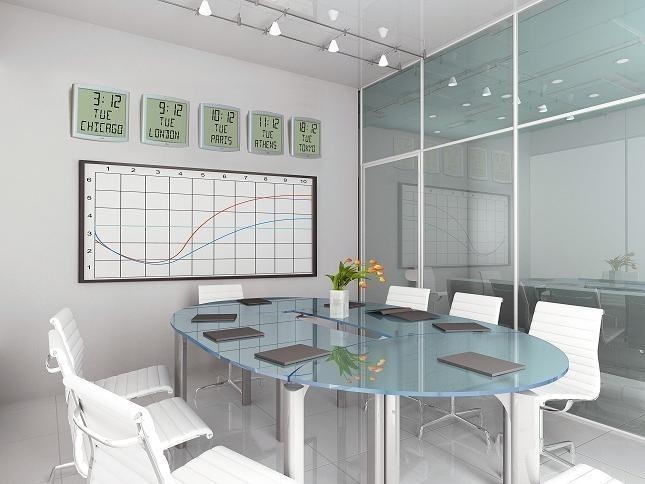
47;330;213;451
497;301;604;469
362;285;433;410
61;375;294;484
464;269;625;343
416;292;502;445
45;308;174;399
411;266;448;312
197;283;266;403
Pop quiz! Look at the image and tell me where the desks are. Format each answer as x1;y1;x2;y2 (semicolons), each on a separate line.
445;274;645;384
169;299;570;484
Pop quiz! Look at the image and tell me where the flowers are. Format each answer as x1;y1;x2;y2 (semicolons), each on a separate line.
608;251;638;271
609;269;616;283
329;342;386;384
327;257;387;290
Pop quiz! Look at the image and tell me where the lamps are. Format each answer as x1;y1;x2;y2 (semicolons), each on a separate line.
328;7;341;21
446;75;575;122
377;25;390;39
197;1;425;78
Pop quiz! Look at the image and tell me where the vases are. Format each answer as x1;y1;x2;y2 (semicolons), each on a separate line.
329;289;351;319
